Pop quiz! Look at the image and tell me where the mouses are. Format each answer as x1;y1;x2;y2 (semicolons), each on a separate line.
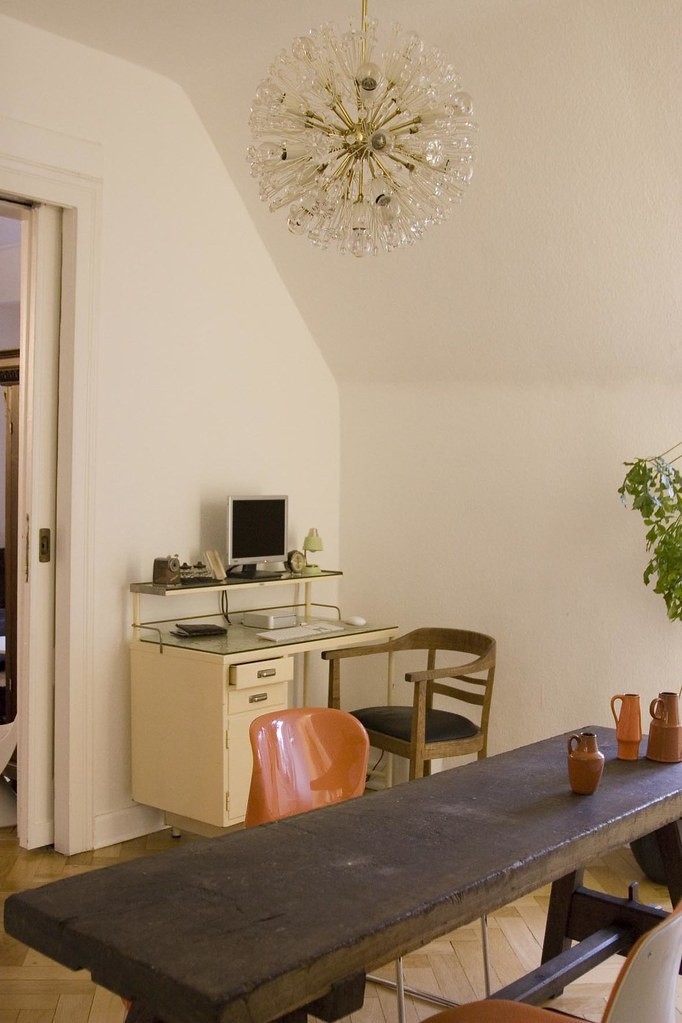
345;616;367;626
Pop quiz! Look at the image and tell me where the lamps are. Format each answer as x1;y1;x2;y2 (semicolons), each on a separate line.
246;0;477;259
302;528;323;576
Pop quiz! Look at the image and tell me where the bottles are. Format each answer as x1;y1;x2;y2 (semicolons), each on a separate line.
611;694;642;761
568;732;605;795
646;692;682;763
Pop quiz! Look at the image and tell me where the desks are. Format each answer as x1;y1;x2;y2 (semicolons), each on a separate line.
4;727;682;1023
132;616;400;838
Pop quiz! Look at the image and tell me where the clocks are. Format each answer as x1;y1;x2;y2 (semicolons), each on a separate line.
283;550;306;574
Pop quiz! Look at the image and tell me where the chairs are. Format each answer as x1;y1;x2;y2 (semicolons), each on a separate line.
320;627;497;782
419;898;682;1023
242;708;371;829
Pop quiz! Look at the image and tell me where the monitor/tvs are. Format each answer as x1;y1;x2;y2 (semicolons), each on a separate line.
227;494;288;580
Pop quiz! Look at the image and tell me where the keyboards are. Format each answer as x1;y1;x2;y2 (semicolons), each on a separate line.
256;623;345;642
